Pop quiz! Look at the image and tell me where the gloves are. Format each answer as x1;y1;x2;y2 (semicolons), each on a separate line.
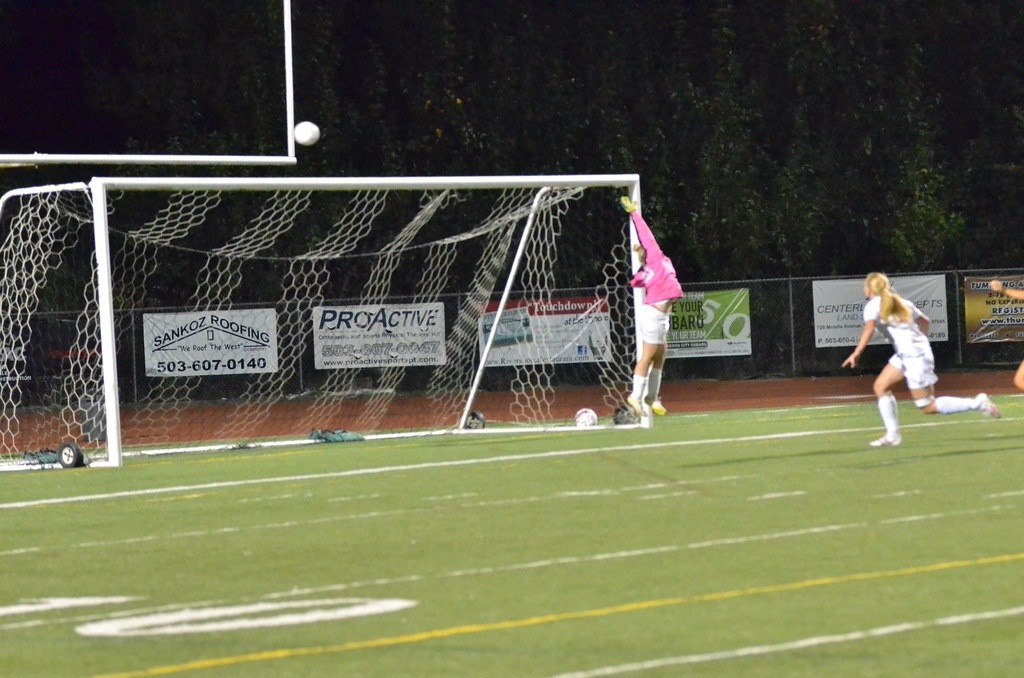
620;196;637;213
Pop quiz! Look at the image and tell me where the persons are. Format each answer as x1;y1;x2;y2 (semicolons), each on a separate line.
842;273;1003;446
619;194;683;420
991;279;1023;393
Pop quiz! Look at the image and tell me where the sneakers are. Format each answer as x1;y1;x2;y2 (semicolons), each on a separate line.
627;395;647;417
870;435;902;447
977;393;1001;418
645;396;666;416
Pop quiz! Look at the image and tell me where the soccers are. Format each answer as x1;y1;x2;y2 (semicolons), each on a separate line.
575;408;597;427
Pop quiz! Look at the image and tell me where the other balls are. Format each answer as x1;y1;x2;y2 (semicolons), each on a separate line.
294;121;320;144
